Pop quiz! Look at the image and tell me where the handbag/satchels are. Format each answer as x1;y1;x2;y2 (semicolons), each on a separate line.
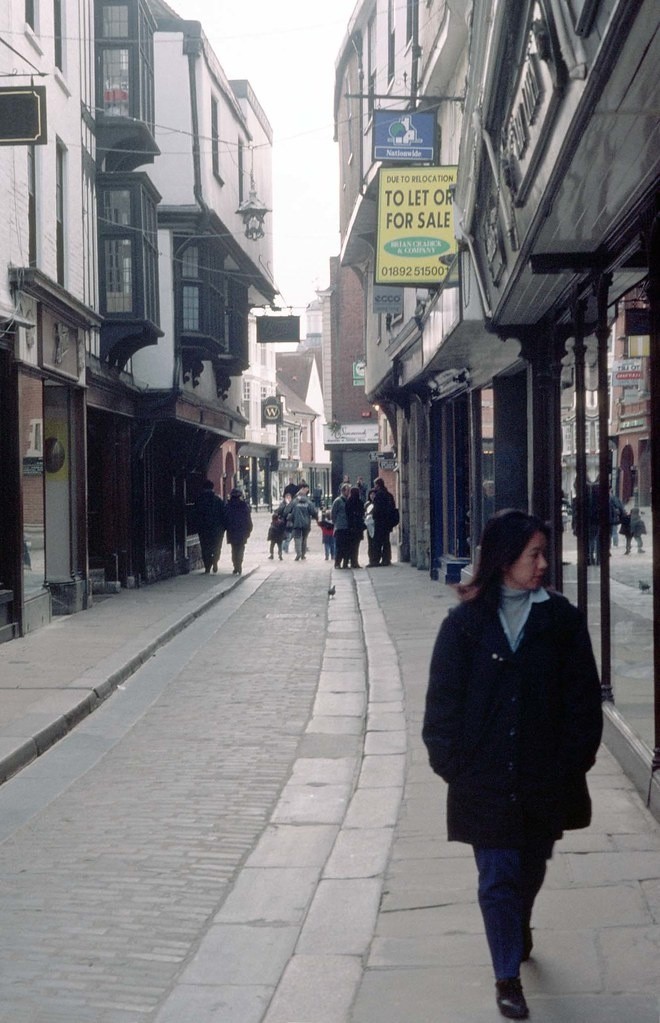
394;509;400;525
286;521;294;533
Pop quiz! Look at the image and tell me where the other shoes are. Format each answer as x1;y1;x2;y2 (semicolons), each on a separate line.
366;560;379;567
381;561;393;566
334;564;341;568
343;564;347;568
351;563;362;569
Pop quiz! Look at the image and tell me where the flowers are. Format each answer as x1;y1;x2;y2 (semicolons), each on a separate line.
328;420;342;435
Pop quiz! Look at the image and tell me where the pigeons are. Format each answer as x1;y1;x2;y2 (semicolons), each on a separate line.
327;585;337;601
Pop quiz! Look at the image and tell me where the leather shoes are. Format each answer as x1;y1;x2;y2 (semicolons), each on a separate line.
494;976;529;1019
523;923;533;960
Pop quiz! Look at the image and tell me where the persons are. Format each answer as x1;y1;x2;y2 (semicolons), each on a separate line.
283;478;298;498
193;480;225;573
316;510;334;560
297;479;309;493
339;475;350;491
226;489;253;574
356;476;367;501
422;509;604;1019
571;475;647;566
313;484;322;507
274;487;318;560
267;514;285;560
363;477;396;567
331;484;365;569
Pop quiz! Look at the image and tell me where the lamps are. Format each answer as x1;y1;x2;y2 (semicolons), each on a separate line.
234;149;273;241
